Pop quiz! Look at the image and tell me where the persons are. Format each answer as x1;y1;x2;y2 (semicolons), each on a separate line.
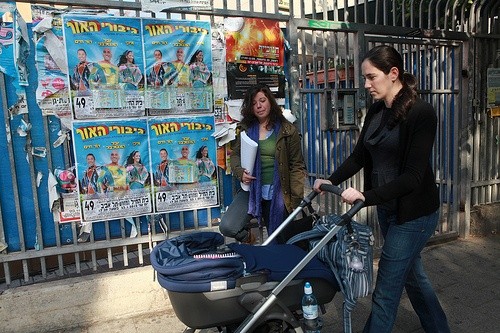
220;83;309;245
79;147;150;195
147;48;212;88
70;45;143;91
313;44;451;333
155;145;215;190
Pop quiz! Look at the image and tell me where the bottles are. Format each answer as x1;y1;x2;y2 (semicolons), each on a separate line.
301;282;318;333
350;255;363;271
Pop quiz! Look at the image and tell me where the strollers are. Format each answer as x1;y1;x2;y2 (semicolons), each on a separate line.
149;184;364;333
